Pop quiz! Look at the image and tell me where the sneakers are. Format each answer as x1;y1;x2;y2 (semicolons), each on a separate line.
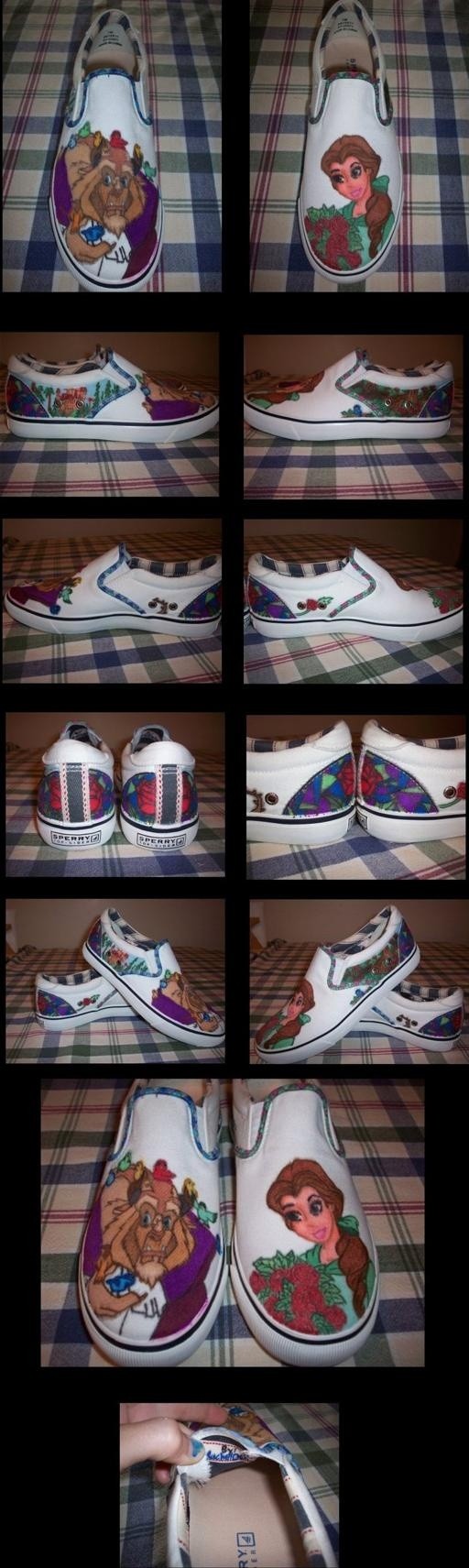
48;9;163;293
120;726;198;851
37;723;118;849
245;350;454;441
4;543;223;639
7;345;219;443
78;1076;228;1368
34;908;225;1047
357;718;466;844
166;1403;337;1568
254;907;463;1063
245;547;463;643
244;722;356;843
298;1;402;282
232;1079;381;1369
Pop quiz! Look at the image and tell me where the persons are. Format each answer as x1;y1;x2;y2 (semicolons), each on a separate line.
321;134;394;270
120;1403;227;1485
265;1158;374;1329
257;979;314;1051
